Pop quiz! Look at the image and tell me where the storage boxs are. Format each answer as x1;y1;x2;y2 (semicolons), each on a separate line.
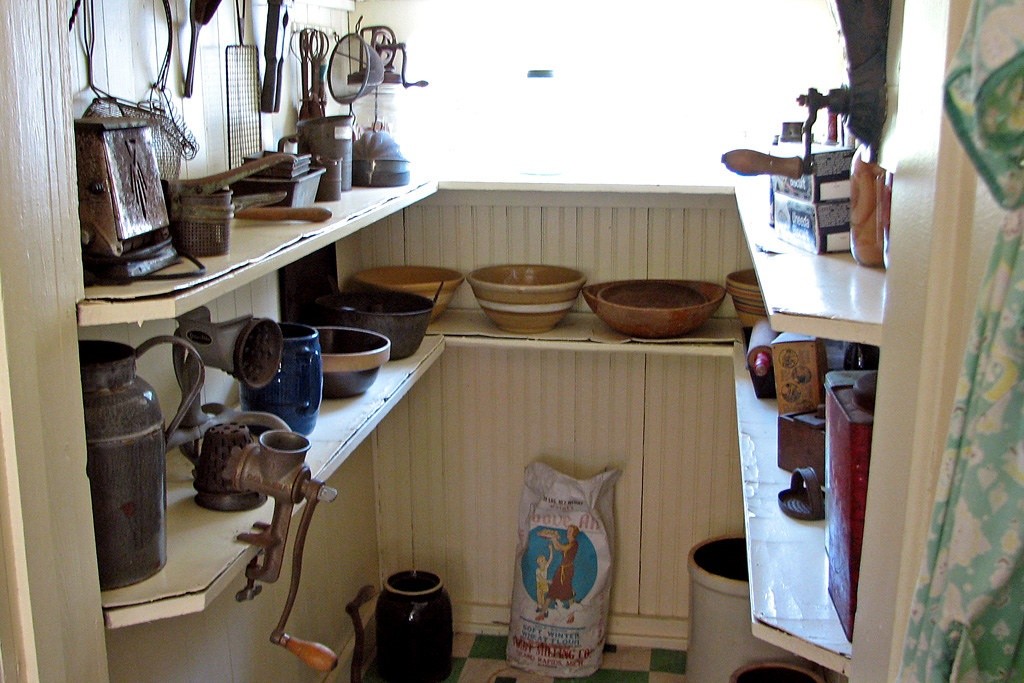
772;332;827;415
769;140;855;253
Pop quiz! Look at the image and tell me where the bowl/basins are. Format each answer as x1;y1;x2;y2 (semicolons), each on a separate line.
724;268;769;328
729;662;825;683
581;279;726;340
312;327;392;400
465;264;588;335
351;266;466;324
314;292;434;361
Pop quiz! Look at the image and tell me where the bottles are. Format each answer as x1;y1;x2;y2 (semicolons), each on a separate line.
769;134;780;229
77;335;206;591
746;319;778;376
376;569;453;683
849;142;883;266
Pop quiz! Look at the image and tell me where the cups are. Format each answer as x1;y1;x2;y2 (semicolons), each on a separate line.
239;322;324;436
296;115;354;201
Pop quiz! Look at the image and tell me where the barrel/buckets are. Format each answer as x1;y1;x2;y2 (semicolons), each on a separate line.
686;533;820;683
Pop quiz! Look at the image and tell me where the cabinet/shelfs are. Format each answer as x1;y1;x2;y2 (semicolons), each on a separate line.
732;174;887;677
76;176;445;628
